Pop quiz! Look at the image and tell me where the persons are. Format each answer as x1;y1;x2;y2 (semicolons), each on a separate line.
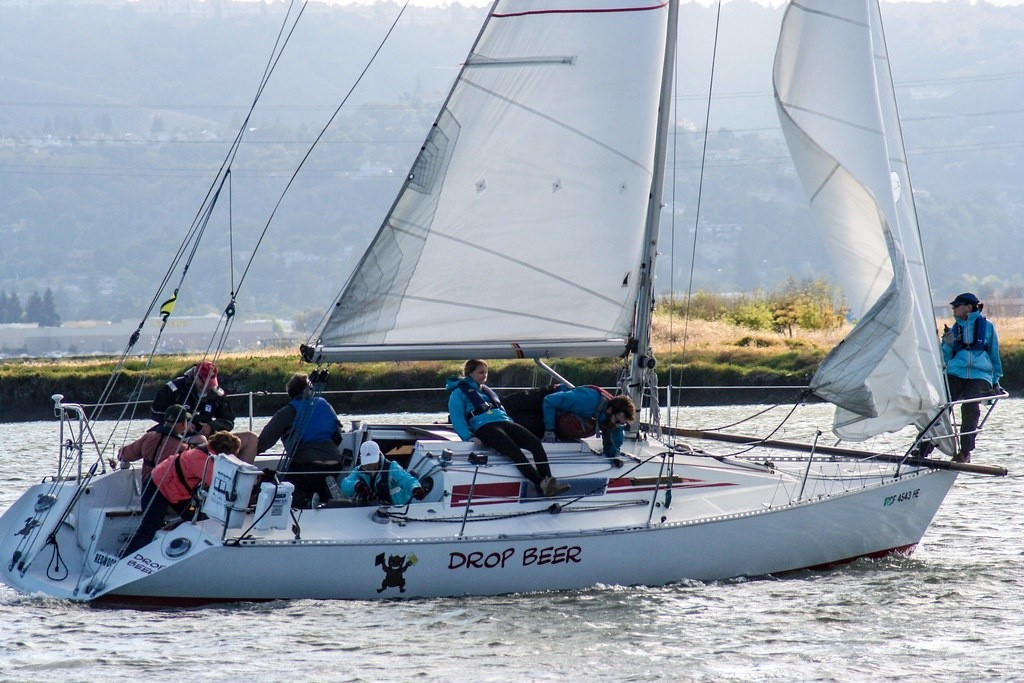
340;441;426;506
257;374;342;508
446;358;572;497
498;386;635;456
911;294;1003;461
117;361;257;556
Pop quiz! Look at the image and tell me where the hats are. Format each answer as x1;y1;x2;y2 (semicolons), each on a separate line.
360;440;379;465
194;360;219;388
163;404;187;423
949;293;979;303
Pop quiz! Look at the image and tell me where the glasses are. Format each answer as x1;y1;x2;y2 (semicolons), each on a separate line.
613;414;624;424
953;302;963;306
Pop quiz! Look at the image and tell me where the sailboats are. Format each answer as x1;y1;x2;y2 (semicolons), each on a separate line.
0;0;1009;609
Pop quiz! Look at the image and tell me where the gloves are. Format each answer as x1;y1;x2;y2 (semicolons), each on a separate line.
612;458;624;468
413;487;424;499
355;481;369;497
540;431;559;442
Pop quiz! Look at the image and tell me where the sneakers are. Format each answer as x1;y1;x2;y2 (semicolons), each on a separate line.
950;451;970;463
540;475;570;497
